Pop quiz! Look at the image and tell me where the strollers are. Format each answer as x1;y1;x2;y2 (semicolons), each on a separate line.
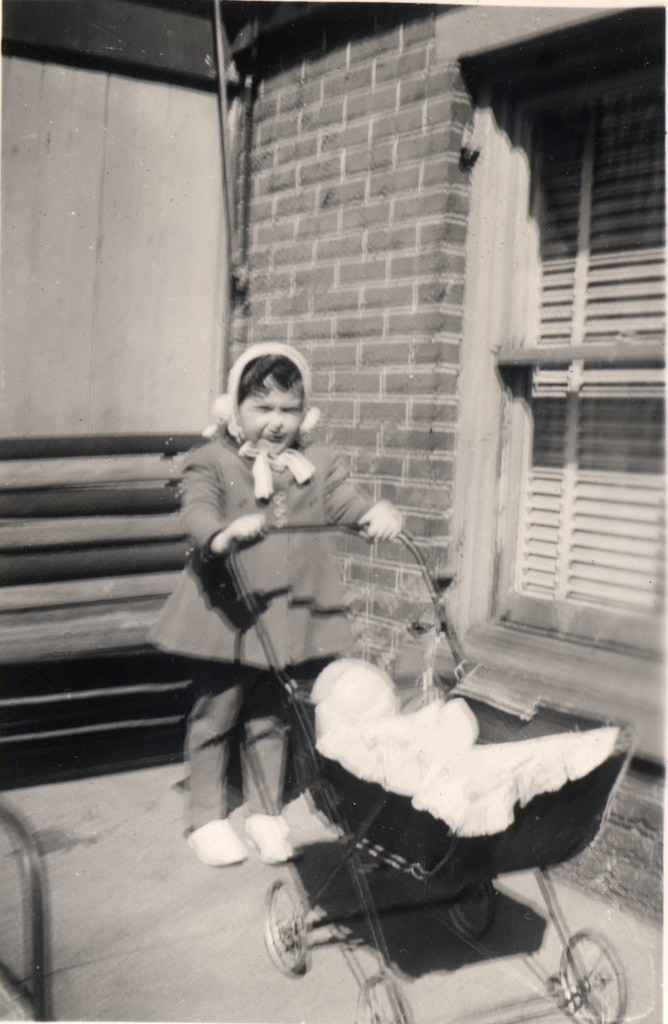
225;523;633;1024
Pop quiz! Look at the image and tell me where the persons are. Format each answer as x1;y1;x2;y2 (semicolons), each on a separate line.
147;342;403;867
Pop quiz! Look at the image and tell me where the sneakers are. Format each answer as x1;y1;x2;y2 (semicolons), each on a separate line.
188;815;249;867
246;811;294;865
